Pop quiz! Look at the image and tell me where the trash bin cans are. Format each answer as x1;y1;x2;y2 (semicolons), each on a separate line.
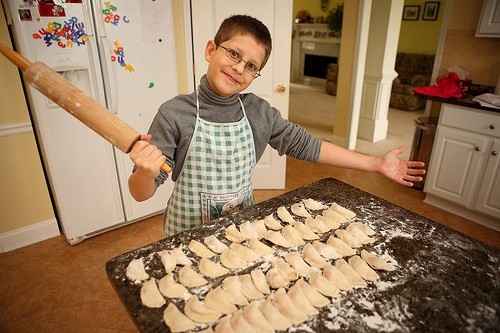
404;116;439;189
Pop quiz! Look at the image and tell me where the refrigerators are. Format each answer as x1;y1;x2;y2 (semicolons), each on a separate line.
2;0;181;246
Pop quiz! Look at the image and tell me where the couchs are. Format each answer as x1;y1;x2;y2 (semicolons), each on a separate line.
326;52;434;112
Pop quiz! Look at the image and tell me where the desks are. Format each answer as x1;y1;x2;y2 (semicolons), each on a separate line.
106;177;500;333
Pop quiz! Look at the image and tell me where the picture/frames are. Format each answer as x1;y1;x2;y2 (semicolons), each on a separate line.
402;5;420;21
422;2;440;21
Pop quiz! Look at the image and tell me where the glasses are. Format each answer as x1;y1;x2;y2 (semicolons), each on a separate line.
216;43;262;78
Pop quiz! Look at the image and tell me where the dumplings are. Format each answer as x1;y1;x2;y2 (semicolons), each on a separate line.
126;199;396;333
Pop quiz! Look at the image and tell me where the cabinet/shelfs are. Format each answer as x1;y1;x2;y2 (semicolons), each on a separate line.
0;4;61;253
291;23;342;84
423;102;500;232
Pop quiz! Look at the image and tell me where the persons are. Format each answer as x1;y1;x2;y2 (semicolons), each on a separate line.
128;14;426;238
